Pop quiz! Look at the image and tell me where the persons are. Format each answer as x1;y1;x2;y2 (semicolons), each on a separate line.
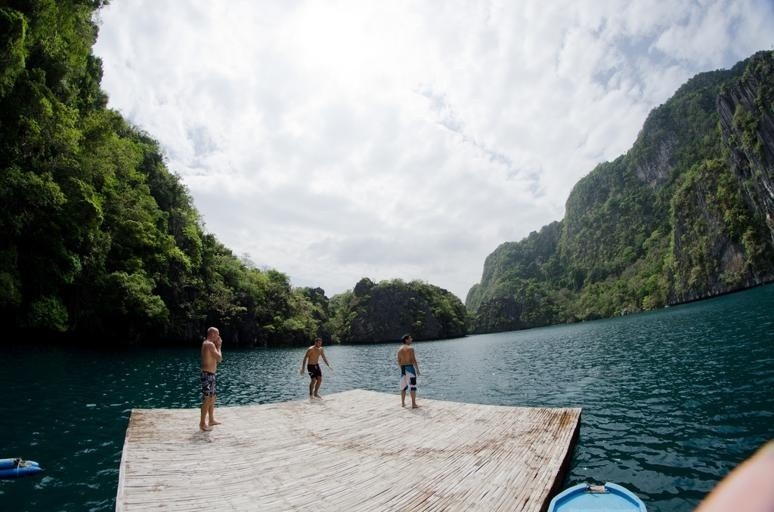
300;337;333;400
396;334;420;408
199;327;223;431
